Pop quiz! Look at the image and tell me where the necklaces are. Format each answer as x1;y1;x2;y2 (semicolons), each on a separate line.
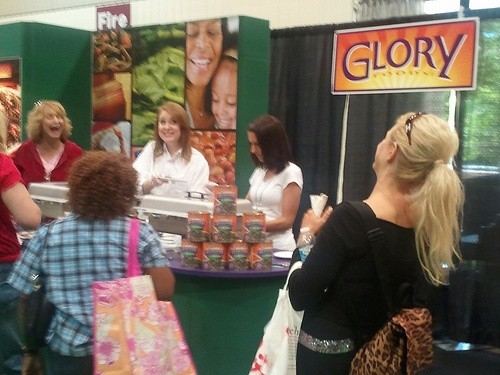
188;93;209;117
255;170;275;208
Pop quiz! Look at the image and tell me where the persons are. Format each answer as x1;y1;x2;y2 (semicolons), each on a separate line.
131;101;210;194
8;99;89;190
8;150;174;375
287;110;459;375
239;112;303;255
205;32;240;131
183;16;230;130
0;101;43;375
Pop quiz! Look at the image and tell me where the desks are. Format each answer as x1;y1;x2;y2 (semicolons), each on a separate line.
14;223;295;375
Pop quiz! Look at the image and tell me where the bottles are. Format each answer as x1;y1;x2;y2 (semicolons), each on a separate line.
297;228;316;263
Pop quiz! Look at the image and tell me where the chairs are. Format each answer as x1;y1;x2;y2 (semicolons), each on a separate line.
432;175;500;353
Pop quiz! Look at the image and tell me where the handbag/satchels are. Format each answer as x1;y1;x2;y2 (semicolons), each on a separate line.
247;260;305;375
21;220;56;349
93;217;196;375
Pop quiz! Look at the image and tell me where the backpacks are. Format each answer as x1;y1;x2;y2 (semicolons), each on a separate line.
346;200;434;375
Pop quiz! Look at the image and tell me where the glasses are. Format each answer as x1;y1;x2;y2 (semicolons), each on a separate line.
405;111;427;146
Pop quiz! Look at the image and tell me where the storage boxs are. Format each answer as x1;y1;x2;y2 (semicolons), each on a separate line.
138;190;252;240
28;182;71;219
